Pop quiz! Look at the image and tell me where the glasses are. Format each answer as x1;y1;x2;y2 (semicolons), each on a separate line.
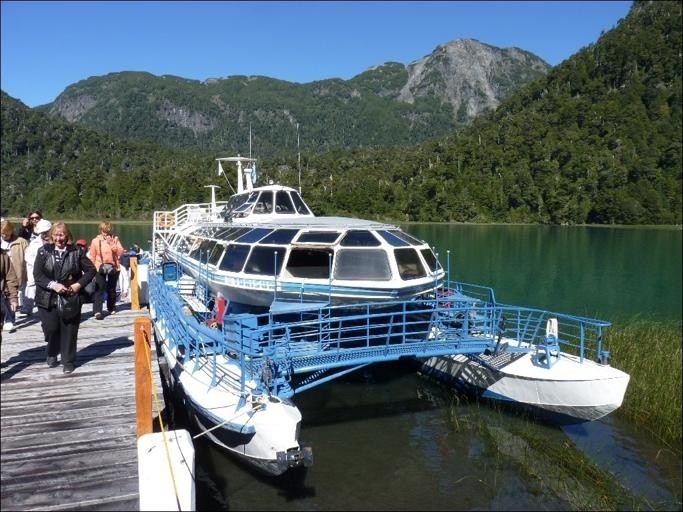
30;217;39;219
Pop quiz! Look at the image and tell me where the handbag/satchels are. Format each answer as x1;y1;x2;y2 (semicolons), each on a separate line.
56;293;80;320
100;263;113;275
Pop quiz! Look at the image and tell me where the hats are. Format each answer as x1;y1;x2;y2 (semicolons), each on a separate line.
33;219;52;234
76;239;87;246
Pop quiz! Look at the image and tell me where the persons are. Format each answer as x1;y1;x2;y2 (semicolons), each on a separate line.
0;211;140;374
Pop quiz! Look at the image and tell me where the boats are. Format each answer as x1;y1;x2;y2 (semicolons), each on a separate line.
148;154;631;479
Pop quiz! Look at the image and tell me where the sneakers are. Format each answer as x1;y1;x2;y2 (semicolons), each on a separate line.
63;363;74;374
47;355;57;367
111;311;116;314
3;322;13;331
94;312;101;319
